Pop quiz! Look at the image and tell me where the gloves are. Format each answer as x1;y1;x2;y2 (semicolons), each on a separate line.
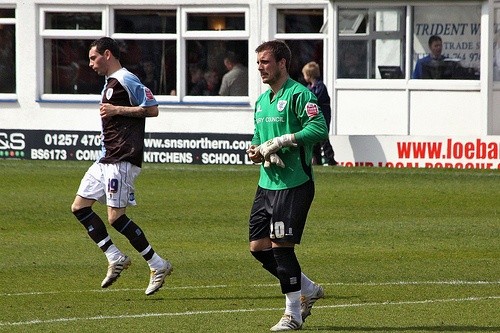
252;134;299;169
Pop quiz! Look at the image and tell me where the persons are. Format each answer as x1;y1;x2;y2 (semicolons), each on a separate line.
341;49;367;79
139;49;248;97
412;35;448;79
246;40;328;331
70;34;173;294
302;62;337;167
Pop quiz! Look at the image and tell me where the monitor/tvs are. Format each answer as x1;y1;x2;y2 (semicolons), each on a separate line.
378;66;403;79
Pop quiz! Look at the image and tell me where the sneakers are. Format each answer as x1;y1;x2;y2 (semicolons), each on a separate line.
270;313;303;331
100;255;131;288
299;283;326;323
143;258;173;297
